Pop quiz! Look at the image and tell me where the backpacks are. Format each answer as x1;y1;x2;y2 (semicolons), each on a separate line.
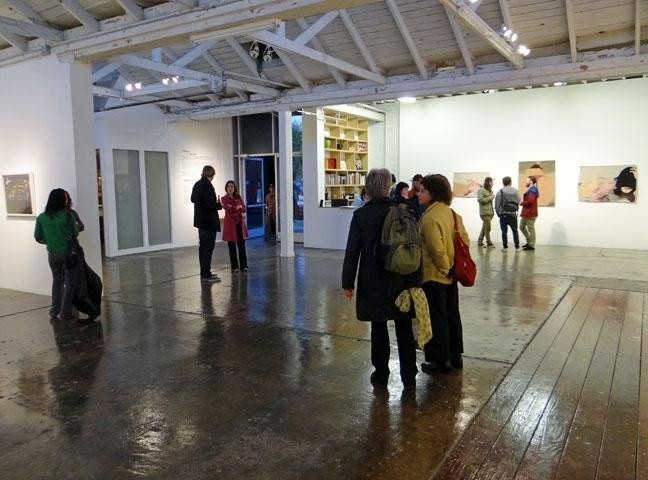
372;203;424;276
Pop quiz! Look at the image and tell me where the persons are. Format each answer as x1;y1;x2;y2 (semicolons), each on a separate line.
297;192;304;208
394;182;421;222
409;174;423;198
222;180;249;273
476;177;496;247
65;190;84;233
390;174;398;199
266;184;276;240
34;189;79;322
417;175;471;376
342;169;424;390
495;177;521;252
519;176;539;251
191;165;222;282
351;188;370;206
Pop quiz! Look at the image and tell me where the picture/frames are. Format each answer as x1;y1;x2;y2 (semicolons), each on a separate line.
518;161;556;207
453;172;492;198
2;174;36;217
578;165;639;204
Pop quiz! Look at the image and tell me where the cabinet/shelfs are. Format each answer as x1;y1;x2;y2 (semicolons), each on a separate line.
323;107;370;209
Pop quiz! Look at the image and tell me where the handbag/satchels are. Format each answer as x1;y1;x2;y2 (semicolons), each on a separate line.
501;189;519;212
66;209;86;270
452;208;476;287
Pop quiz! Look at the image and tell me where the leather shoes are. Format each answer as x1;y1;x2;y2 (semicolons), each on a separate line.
451;358;463;371
422;362;450;373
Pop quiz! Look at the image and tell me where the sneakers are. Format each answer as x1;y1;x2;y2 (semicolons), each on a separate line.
211;273;218;277
371;372;387;390
523;245;535;251
522;244;528;248
402;378;417;386
202;277;221;282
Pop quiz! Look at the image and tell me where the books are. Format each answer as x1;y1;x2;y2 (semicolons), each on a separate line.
324;173;366;185
